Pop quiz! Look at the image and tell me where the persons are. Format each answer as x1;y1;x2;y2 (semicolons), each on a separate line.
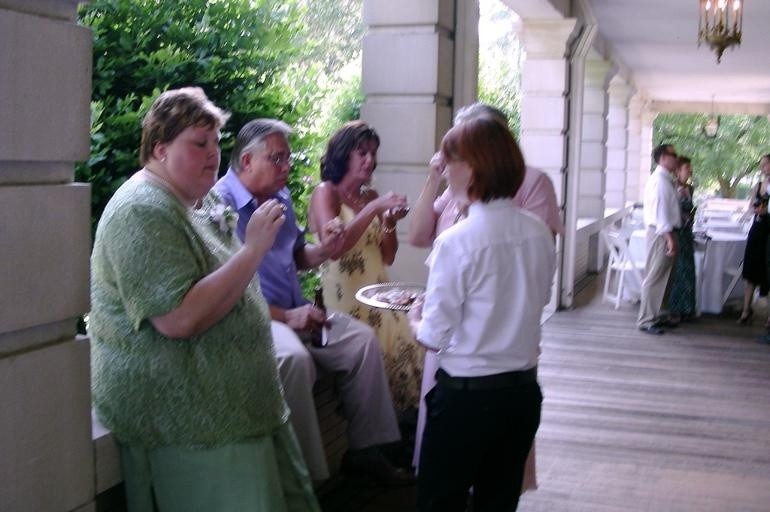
205;118;402;484
307;121;426;415
409;102;560;248
405;119;556;511
664;155;696;322
636;144;682;335
736;153;770;327
90;86;319;512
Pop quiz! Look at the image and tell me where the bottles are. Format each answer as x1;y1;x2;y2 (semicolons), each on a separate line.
311;287;328;349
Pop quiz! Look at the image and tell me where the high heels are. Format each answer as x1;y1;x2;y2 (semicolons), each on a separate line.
765;316;770;327
736;307;753;326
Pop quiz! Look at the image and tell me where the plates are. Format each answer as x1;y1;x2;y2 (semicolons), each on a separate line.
355;282;427;309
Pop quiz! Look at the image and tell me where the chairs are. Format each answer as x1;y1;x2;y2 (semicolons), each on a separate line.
599;230;646;311
722;261;746;314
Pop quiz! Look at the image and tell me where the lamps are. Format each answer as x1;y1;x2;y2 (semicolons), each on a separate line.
704;93;721;138
697;1;742;65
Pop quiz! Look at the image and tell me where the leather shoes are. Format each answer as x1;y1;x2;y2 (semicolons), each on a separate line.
642;325;662;333
660;318;673;326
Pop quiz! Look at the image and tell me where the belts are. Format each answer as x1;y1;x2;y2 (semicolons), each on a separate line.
436;368;537;384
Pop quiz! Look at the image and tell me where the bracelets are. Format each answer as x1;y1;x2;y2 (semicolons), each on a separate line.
381;224;398;235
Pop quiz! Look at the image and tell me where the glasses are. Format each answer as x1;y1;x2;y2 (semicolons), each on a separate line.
252;155;293;168
665;151;677;158
